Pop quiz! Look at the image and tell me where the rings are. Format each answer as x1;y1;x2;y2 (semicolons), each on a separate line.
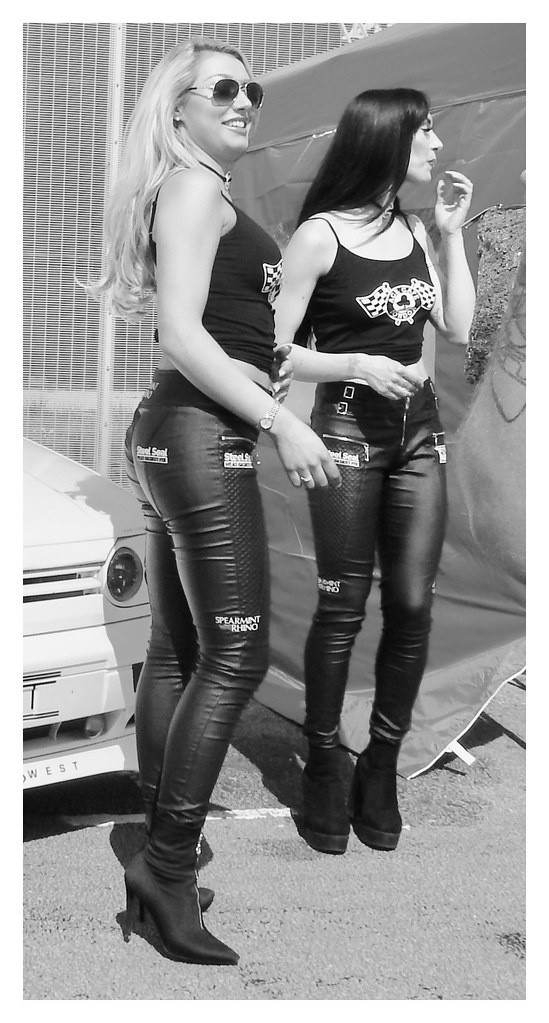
301;475;312;481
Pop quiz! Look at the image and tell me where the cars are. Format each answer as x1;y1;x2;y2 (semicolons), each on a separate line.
22;437;152;789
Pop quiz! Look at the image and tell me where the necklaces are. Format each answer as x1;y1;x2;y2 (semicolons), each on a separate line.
198;159;232;190
374;200;395;218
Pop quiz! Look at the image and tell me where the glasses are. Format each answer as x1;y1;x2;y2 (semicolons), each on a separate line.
184;79;264;109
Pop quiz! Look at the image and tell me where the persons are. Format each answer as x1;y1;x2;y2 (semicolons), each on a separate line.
273;88;477;856
77;37;343;965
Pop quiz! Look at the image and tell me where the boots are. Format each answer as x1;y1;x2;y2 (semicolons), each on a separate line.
123;812;240;965
351;736;402;849
304;728;351;854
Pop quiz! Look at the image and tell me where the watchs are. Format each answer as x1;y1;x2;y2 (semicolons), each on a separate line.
259;401;280;432
